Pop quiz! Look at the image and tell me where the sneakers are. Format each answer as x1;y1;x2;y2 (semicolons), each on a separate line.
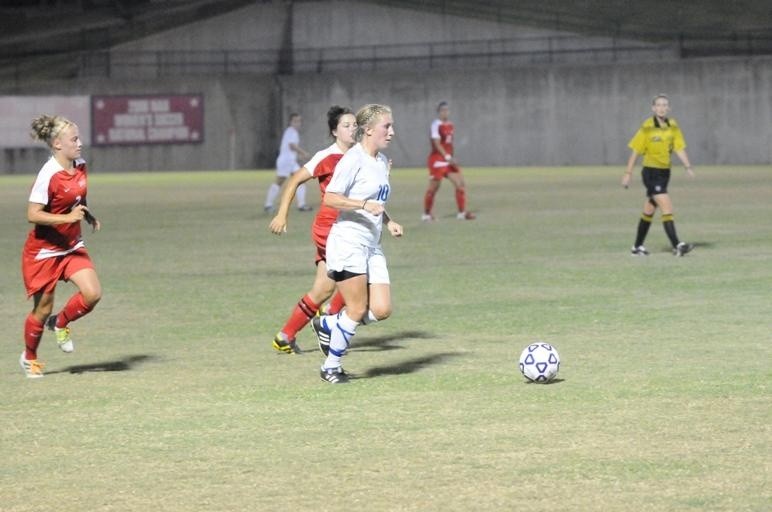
456;210;477;221
672;241;694;257
319;363;354;385
421;214;437;222
45;314;76;354
630;245;649;256
297;205;312;211
18;350;46;380
310;314;332;359
314;303;330;319
271;330;306;356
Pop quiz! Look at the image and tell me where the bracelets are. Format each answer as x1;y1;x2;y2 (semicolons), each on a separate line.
623;171;633;176
684;163;691;170
361;200;368;209
444;153;453;162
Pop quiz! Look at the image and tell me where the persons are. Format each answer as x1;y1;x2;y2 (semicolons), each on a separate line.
620;92;695;257
310;102;404;383
18;114;103;380
265;111;316;214
419;99;476;221
265;105;393;356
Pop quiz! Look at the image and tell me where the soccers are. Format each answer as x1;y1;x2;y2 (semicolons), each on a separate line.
521;341;559;383
